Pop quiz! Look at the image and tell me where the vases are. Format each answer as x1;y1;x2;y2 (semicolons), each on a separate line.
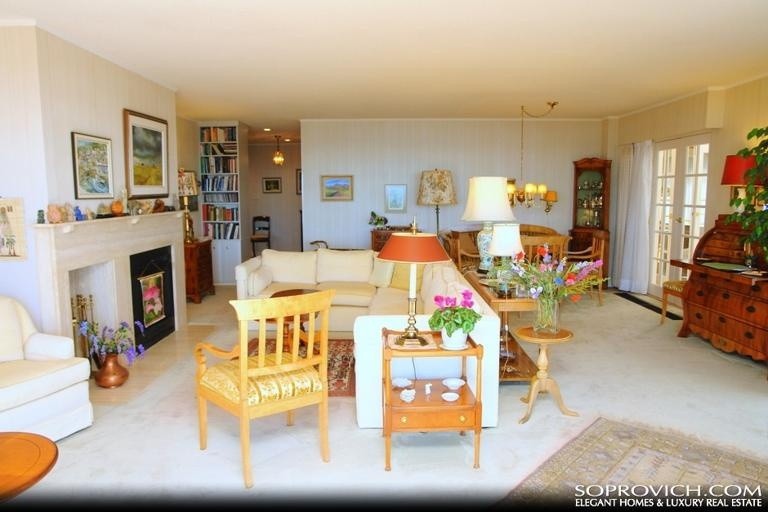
377;221;384;230
533;298;561;335
439;326;468;350
95;352;129;389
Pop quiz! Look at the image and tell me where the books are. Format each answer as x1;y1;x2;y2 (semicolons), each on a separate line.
200;126;239;240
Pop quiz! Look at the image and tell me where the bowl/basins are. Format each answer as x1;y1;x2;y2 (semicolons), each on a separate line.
441;392;460;402
441;378;466;390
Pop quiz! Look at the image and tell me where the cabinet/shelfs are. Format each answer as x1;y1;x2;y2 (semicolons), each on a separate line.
671;214;768;363
199;121;249;285
370;226;422;252
184;239;215;303
570;157;612;290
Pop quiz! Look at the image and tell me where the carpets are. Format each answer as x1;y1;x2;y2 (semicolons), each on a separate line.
229;338;356;397
490;413;768;511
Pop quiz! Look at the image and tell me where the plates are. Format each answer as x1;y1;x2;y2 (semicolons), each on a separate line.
392;376;412;389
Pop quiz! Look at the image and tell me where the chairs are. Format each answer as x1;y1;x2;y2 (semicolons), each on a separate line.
660;280;689;324
250;216;270;257
0;295;94;442
439;224;605;306
194;287;336;489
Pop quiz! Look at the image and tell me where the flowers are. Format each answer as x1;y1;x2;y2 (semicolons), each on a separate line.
428;289;482;338
77;319;145;367
368;211;388;225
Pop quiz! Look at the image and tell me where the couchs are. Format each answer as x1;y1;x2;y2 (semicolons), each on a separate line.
235;255;501;428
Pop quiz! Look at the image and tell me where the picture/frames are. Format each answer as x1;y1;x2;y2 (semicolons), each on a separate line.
71;132;114;199
296;169;301;195
123;108;169;200
320;175;353;202
262;177;282;193
384;184;407;214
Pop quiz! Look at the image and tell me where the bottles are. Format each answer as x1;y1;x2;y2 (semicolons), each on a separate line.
425;383;432;395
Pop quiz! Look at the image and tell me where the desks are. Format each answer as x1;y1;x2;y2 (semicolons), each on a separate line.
465;270;557;382
0;431;58;504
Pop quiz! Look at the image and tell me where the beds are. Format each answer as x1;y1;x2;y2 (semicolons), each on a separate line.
381;327;483;471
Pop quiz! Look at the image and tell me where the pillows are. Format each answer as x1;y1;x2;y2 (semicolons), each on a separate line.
248;248;483;316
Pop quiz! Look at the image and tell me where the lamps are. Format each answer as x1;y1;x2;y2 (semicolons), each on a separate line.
417;168;457;239
374;215;452;346
720;154;768;213
178;171;200;244
461;176;517;274
272;136;285;166
506;102;559;212
